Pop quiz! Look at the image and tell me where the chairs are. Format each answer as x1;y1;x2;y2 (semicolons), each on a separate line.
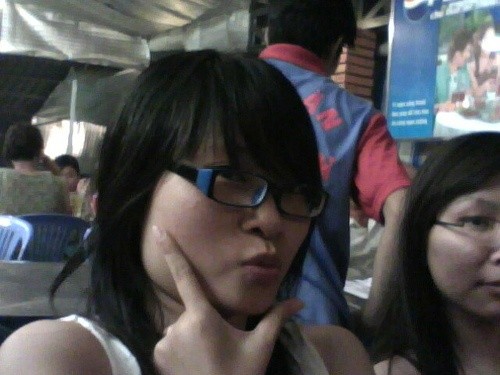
0;211;94;261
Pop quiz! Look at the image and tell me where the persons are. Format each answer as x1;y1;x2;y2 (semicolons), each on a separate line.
0;124;92;218
259;0;410;346
346;198;383;282
374;132;500;375
435;26;500;112
0;49;374;375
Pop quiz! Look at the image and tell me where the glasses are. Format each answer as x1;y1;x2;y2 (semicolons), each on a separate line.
162;158;329;218
435;216;500;238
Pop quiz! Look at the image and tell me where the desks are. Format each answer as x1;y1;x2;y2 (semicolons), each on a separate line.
434;110;500;138
0;263;98;328
